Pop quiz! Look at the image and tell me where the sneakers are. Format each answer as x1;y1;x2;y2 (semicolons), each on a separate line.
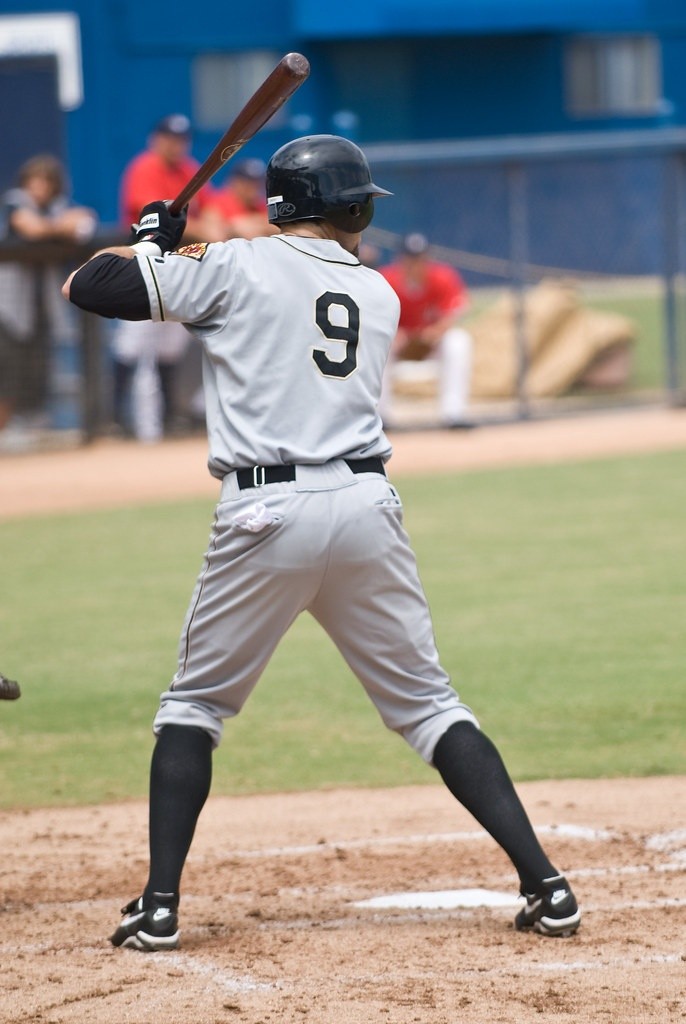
111;893;180;951
515;875;581;938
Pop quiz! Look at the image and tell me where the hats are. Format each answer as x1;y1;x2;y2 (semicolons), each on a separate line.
154;114;193;142
402;232;429;257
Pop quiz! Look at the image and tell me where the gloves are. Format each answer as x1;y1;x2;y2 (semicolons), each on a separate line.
130;198;189;258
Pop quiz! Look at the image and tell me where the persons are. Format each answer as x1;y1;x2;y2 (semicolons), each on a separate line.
61;132;581;954
0;110;478;454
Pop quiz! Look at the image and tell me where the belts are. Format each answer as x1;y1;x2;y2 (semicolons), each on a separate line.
237;457;386;490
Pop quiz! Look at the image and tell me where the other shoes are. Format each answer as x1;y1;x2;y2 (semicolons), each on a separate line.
0;674;21;701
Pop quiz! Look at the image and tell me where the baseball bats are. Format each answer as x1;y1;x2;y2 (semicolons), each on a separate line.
168;53;309;213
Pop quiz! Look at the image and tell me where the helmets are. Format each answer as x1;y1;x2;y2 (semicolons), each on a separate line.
265;134;393;234
